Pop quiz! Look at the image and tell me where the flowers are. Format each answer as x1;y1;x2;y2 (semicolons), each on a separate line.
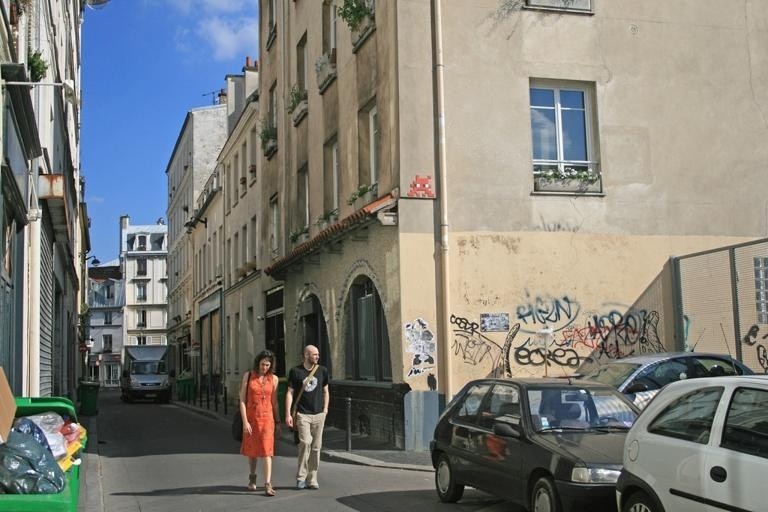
534;166;602;184
289;183;377;243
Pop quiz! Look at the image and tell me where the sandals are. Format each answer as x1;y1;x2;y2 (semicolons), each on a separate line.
248;480;257;491
265;487;276;496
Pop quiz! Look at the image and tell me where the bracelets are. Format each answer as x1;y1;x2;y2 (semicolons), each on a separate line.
275;421;280;424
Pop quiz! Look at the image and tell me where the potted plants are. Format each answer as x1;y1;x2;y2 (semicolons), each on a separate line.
334;0;375;46
258;83;309;157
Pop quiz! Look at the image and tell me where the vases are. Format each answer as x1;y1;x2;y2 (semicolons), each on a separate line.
294;232;309;247
535;177;601;193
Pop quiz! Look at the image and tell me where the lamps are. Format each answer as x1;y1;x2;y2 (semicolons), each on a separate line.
83;255;100;266
183;216;207;234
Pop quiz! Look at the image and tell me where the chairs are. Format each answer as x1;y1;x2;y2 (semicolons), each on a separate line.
558;402;591;429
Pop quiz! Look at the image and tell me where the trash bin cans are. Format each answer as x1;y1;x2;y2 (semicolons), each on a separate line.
178;372;194;401
80;381;100;417
0;396;87;512
277;377;290;422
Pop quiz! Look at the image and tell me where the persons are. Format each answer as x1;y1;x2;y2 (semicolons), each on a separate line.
285;344;330;489
239;350;282;497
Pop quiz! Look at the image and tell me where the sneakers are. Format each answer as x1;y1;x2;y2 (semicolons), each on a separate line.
307;481;319;489
297;480;305;489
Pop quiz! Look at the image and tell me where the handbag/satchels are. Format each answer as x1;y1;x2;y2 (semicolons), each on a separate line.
292;423;299;446
231;410;244;442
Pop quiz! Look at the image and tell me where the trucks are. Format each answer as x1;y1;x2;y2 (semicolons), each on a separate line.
120;344;172;404
